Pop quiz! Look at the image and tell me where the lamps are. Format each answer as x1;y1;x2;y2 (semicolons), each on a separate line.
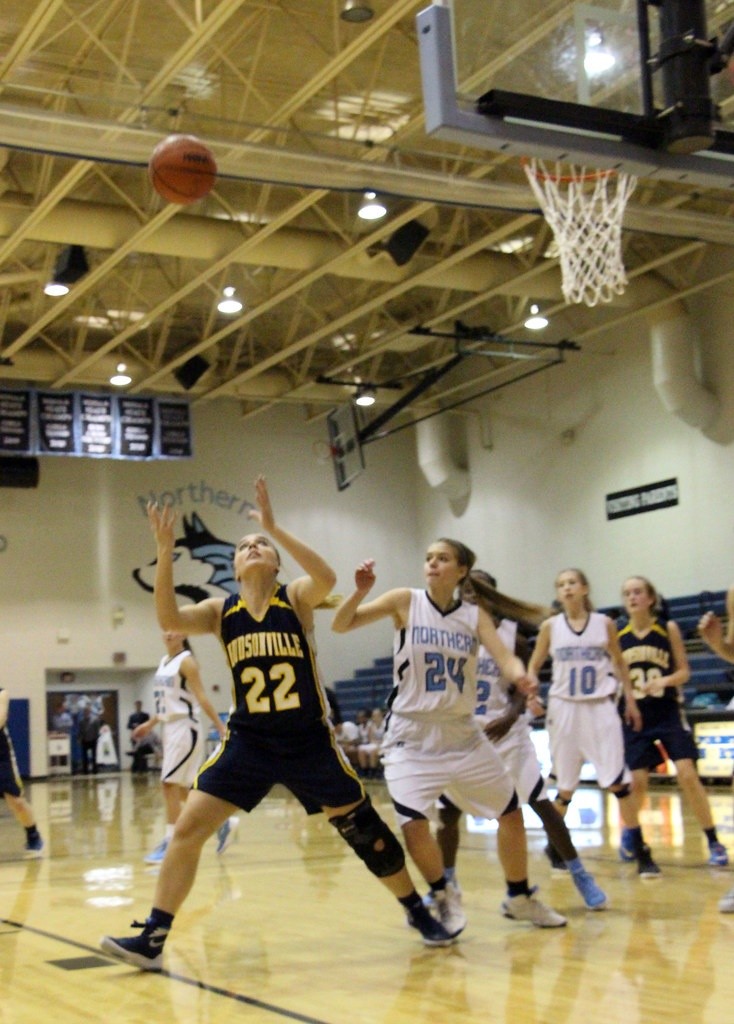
216;285;244;313
357;192;386;220
340;0;373;22
524;304;548;329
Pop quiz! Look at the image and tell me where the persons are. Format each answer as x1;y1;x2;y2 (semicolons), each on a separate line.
101;474;451;970
0;687;45;853
698;586;734;911
127;700;149;774
77;707;101;776
132;632;240;863
336;708;385;778
526;569;662;878
421;569;607;910
612;577;728;867
329;536;568;938
52;703;73;766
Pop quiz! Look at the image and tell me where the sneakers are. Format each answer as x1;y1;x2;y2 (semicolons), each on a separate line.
405;901;452;946
619;847;637;862
572;871;607;909
144;837;170;863
638;848;661;878
422;879;461;908
428;884;466;938
709;841;728;865
499;885;567;928
216;816;239;853
101;917;169;972
544;849;569;874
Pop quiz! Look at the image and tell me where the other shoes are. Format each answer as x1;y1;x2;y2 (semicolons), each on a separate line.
21;839;43;859
719;889;734;912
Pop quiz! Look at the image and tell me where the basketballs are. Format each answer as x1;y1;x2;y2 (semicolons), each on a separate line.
148;134;218;206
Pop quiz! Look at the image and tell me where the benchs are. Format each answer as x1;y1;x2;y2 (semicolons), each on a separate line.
330;591;734;724
